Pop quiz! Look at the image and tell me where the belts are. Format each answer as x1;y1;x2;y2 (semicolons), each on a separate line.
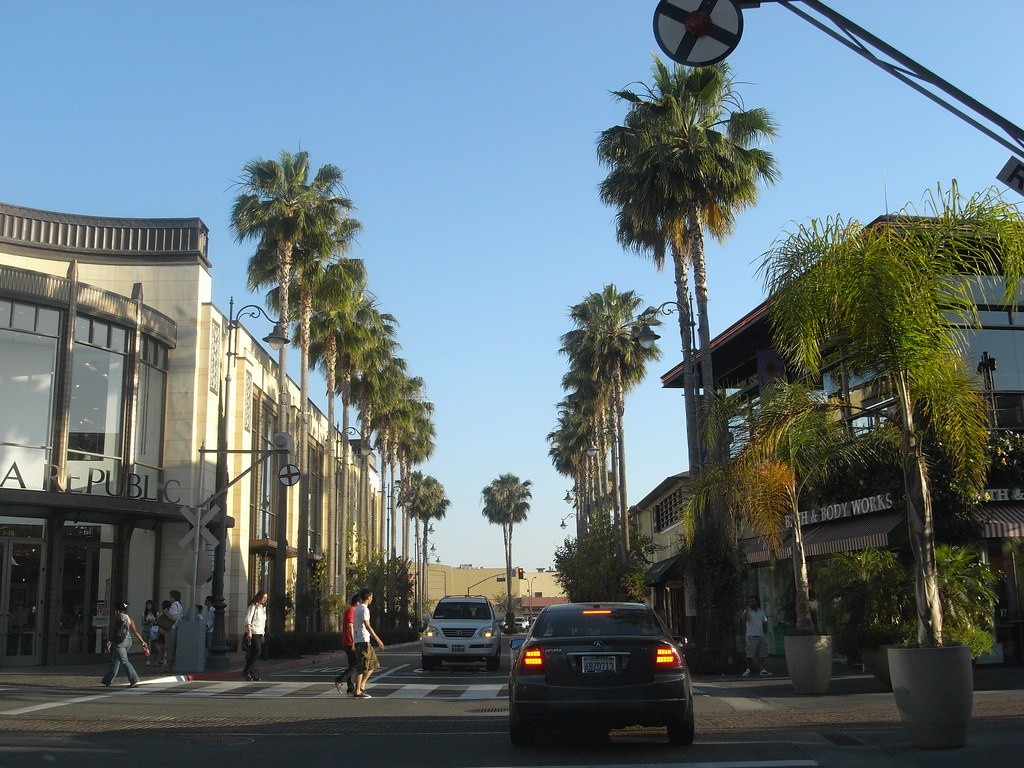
254;634;265;637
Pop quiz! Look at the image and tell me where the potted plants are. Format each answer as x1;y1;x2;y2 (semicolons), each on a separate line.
671;186;1024;752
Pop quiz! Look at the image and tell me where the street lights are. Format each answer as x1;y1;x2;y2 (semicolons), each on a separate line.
559;481;587;544
635;301;722;676
208;306;292;668
334;427;377;632
386;485;413;575
524;577;538;625
413;516;442;636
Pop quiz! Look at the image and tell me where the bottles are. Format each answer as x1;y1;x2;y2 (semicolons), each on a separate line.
143;644;150;656
247;637;251;645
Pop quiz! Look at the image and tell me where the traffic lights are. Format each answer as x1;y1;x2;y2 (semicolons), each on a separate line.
518;568;523;580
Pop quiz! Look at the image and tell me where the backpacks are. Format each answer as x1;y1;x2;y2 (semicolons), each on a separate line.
112;614;129;644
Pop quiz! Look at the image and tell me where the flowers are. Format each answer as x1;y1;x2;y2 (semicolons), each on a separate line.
796;475;849;507
989;430;1024;488
835;456;875;496
941;466;997;544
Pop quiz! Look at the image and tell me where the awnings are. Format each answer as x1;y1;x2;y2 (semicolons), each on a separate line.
956;499;1024;537
738;509;911;564
645;555;693;587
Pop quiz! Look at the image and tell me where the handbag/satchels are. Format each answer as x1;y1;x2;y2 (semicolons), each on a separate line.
150;626;159;640
157;613;177;631
242;632;251;651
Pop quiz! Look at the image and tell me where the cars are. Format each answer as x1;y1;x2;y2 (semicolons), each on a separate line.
494;615;527;631
507;600;695;748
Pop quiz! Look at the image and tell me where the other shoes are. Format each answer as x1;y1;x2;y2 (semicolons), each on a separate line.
160;659;167;665
241;671;261;681
153;662;159;665
145;661;151;665
127;680;139;688
100;681;110;686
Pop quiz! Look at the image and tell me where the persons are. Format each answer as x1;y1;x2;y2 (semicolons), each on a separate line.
335;589;386;699
99;600;148;688
242;590;269;681
141;590;215;676
741;595;774;678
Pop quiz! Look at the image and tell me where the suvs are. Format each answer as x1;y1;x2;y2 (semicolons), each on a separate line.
421;595;505;672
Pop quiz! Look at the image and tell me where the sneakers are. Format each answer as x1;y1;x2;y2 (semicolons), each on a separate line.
760;672;773;676
742;671;753;676
347;689;354;695
335;678;343;694
351;688;372;699
